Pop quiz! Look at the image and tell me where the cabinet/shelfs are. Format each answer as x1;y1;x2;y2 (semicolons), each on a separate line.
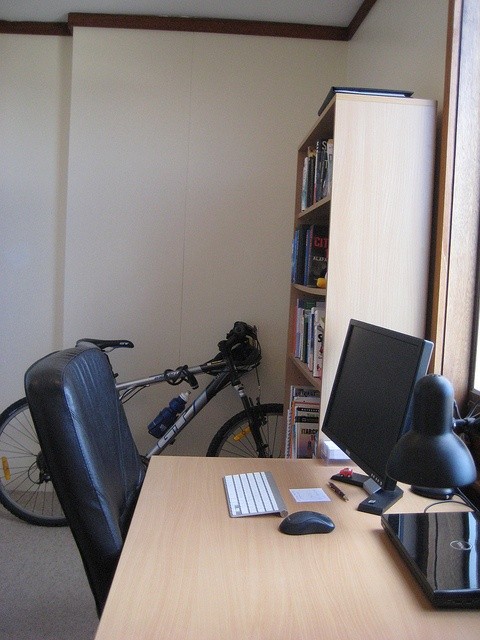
282;93;439;458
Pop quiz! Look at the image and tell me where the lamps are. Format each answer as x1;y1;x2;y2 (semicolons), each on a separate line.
387;373;477;489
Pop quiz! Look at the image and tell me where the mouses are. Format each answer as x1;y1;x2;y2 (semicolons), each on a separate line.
282;510;334;535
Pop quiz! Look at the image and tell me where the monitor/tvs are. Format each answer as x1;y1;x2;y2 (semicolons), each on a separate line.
320;316;434;514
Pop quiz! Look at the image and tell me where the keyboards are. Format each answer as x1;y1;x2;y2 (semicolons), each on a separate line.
223;471;286;519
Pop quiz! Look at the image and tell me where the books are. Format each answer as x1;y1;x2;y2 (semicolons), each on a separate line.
318;86;414;117
290;224;326;288
301;139;334;211
289;297;326;378
285;385;321;459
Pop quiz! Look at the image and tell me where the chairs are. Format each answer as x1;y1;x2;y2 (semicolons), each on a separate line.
24;347;146;622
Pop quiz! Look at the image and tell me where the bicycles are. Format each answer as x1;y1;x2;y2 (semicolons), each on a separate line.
0;321;284;527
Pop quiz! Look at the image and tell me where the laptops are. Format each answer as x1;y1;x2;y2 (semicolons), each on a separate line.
382;514;479;608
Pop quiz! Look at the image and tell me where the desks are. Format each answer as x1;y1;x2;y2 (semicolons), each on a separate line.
94;455;480;640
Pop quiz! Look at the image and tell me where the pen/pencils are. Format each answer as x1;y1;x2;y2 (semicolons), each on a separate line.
328;482;349;502
